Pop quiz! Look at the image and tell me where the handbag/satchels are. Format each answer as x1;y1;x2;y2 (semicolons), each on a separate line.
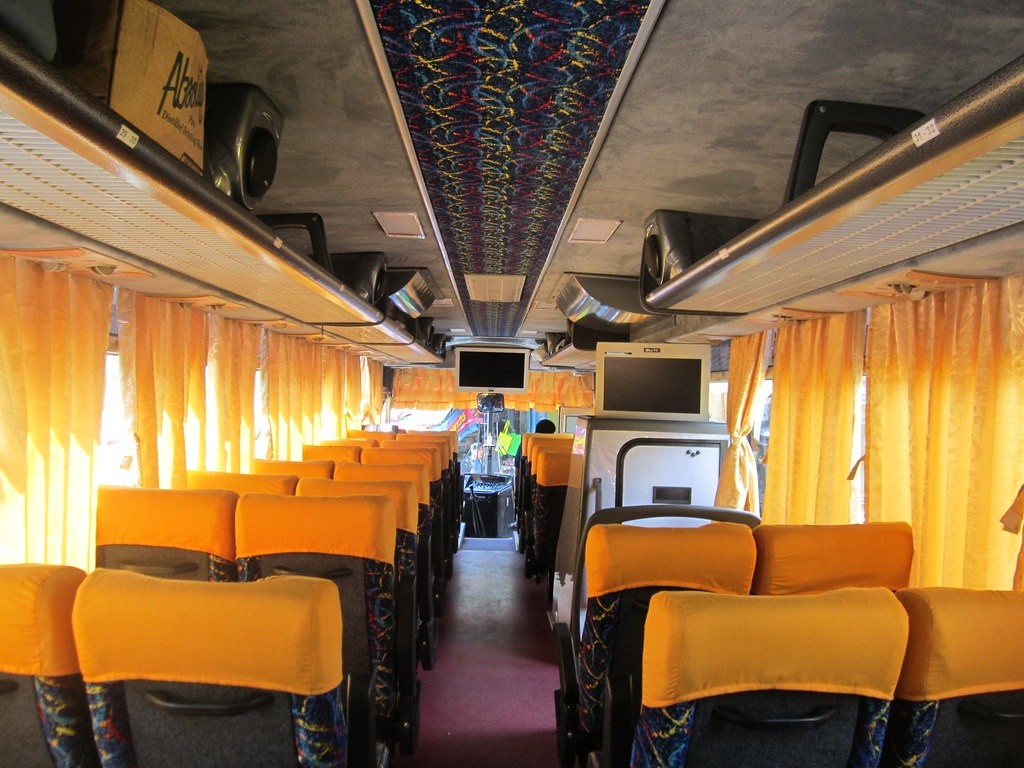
494;421;521;456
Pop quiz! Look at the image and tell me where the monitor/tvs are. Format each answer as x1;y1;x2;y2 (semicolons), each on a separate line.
596;342;711;422
454;347;529;393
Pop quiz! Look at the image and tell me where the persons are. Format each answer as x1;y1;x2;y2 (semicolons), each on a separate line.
507;419;555;531
458;442;485;487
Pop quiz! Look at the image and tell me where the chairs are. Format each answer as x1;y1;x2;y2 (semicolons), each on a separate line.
880;584;1024;768
585;587;910;768
512;432;576;604
751;521;916;593
0;433;469;767
549;522;758;768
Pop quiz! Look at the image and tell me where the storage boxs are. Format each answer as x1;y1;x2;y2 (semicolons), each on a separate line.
52;0;209;179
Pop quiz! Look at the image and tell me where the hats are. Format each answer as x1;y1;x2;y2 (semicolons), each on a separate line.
470;442;483;451
